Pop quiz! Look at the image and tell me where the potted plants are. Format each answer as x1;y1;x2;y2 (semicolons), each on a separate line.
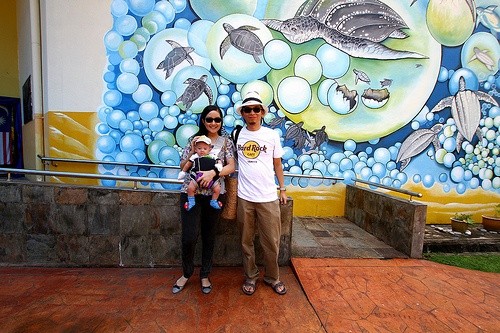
482;206;500;234
450;212;472;233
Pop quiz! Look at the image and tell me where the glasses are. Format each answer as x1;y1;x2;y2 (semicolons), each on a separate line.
204;117;222;123
241;106;263;114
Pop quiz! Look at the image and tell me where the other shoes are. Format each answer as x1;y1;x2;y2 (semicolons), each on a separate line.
172;276;212;294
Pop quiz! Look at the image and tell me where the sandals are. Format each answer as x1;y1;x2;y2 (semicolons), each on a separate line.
241;275;287;295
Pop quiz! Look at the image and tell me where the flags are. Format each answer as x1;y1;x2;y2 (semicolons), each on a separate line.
0;114;14;166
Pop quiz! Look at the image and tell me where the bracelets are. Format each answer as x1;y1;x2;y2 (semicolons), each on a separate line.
280;186;286;191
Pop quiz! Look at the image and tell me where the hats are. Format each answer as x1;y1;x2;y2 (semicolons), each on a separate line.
236;91;269;118
194;135;212;145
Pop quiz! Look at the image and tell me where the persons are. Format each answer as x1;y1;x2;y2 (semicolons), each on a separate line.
229;94;289;296
170;103;238;294
176;134;224;211
